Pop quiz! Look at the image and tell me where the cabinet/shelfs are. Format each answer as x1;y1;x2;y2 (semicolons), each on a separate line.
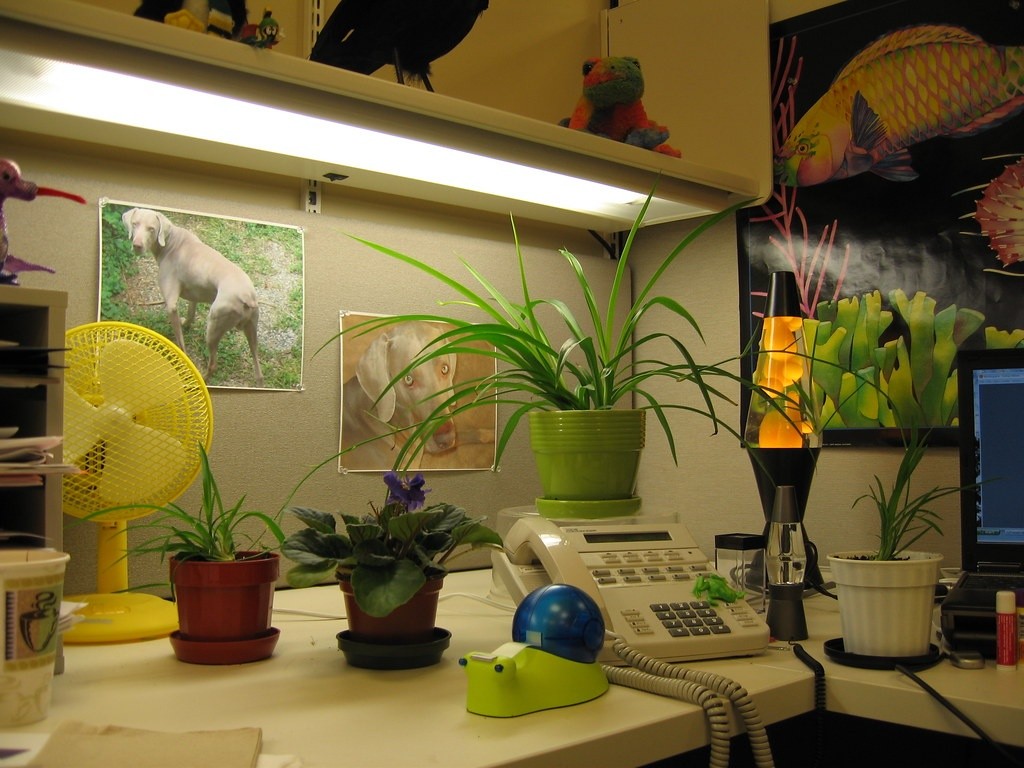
0;284;69;675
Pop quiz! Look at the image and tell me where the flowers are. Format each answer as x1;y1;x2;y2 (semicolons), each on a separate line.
279;470;512;619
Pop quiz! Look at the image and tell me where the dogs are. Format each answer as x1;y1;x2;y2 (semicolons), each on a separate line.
342;320;455;469
122;207;265;388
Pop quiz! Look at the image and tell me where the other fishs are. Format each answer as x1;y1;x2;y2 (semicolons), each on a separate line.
772;26;1024;186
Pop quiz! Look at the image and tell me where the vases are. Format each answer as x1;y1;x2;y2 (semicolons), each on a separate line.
333;565;448;640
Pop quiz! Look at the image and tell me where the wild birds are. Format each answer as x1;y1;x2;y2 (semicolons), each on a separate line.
0;157;88;280
310;0;488;93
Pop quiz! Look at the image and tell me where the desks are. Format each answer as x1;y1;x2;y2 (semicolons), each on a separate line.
1;551;1024;768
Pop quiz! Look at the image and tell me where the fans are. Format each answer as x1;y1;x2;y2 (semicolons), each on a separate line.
59;320;216;645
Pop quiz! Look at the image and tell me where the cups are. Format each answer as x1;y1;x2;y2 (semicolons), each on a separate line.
0;548;71;728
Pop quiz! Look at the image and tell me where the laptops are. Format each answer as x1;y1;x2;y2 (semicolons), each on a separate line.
940;348;1024;657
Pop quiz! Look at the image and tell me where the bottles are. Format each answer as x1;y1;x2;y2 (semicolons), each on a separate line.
996;587;1024;672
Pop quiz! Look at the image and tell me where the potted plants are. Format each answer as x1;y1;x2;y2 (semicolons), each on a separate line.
310;165;907;517
55;436;286;662
823;410;1016;671
